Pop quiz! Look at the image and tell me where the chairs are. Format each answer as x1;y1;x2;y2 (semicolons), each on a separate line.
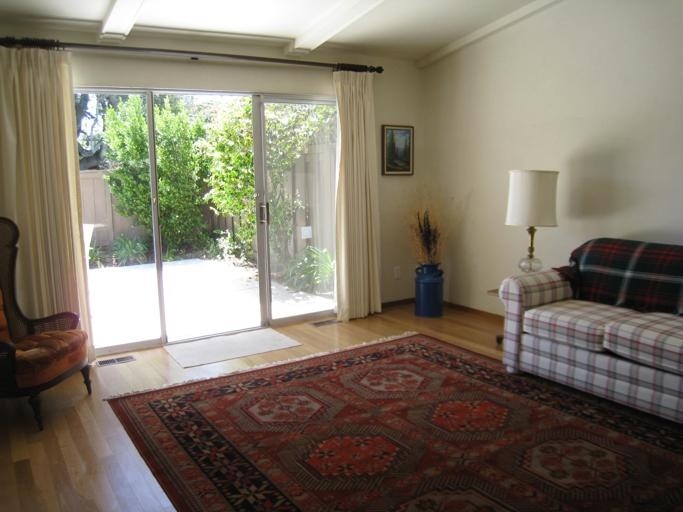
0;215;91;430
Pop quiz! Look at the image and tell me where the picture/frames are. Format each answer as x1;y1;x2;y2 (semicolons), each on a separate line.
382;124;414;175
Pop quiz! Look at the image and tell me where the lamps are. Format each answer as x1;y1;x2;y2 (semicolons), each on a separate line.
98;0;142;41
281;0;383;58
504;170;560;273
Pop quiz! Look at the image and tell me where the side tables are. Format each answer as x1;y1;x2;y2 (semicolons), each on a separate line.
486;289;504;345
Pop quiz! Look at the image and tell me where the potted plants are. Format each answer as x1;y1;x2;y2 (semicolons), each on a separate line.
89;240;108;269
112;233;149;266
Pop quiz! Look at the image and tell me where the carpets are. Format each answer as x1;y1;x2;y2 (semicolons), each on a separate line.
102;329;682;512
163;327;303;369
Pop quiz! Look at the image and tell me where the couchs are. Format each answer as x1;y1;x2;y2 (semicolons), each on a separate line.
498;238;683;428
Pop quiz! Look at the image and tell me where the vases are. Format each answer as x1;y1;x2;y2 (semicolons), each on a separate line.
415;264;443;317
